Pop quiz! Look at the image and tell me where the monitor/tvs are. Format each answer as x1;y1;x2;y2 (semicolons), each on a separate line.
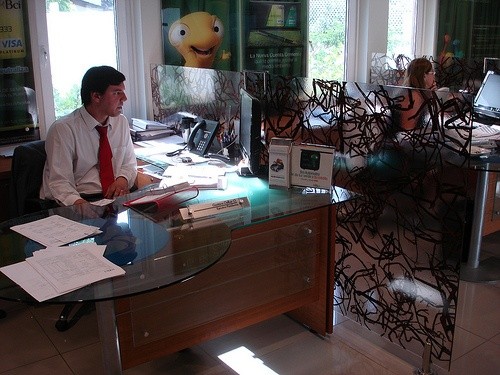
472;70;500;125
238;88;262;176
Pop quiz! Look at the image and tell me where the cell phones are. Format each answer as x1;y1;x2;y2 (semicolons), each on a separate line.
182;157;192;162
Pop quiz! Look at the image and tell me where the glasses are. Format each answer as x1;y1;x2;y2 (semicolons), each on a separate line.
426;70;436;78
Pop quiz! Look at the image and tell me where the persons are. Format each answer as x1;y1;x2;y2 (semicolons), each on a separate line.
39;65;138;232
58;206;137;265
390;57;437;131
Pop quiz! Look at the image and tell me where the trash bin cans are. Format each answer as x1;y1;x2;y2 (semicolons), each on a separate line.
438;199;475;263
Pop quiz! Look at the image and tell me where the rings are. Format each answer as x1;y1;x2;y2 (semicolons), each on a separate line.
121;190;123;191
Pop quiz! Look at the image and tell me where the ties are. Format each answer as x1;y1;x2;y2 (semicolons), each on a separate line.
95;125;115;197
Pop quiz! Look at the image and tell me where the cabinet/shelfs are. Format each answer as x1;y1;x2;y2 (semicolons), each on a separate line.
259;100;500;282
0;139;365;375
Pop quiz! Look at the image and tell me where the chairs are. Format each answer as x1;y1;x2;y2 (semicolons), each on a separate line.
9;140;90;333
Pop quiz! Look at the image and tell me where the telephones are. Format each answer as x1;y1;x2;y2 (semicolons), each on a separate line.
187;118;219;156
